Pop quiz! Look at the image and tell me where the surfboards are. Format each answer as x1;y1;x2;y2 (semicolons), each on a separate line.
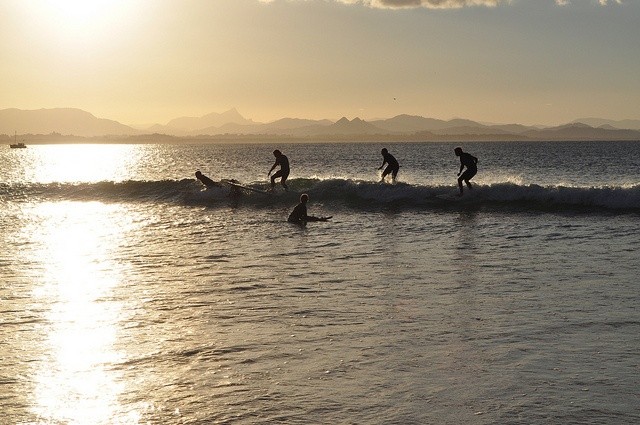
224;181;270;195
434;193;464;202
307;214;333;221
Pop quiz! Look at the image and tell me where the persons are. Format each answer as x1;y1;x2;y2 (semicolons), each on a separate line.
377;148;399;185
195;171;219;189
455;147;478;196
287;193;309;224
267;149;289;191
